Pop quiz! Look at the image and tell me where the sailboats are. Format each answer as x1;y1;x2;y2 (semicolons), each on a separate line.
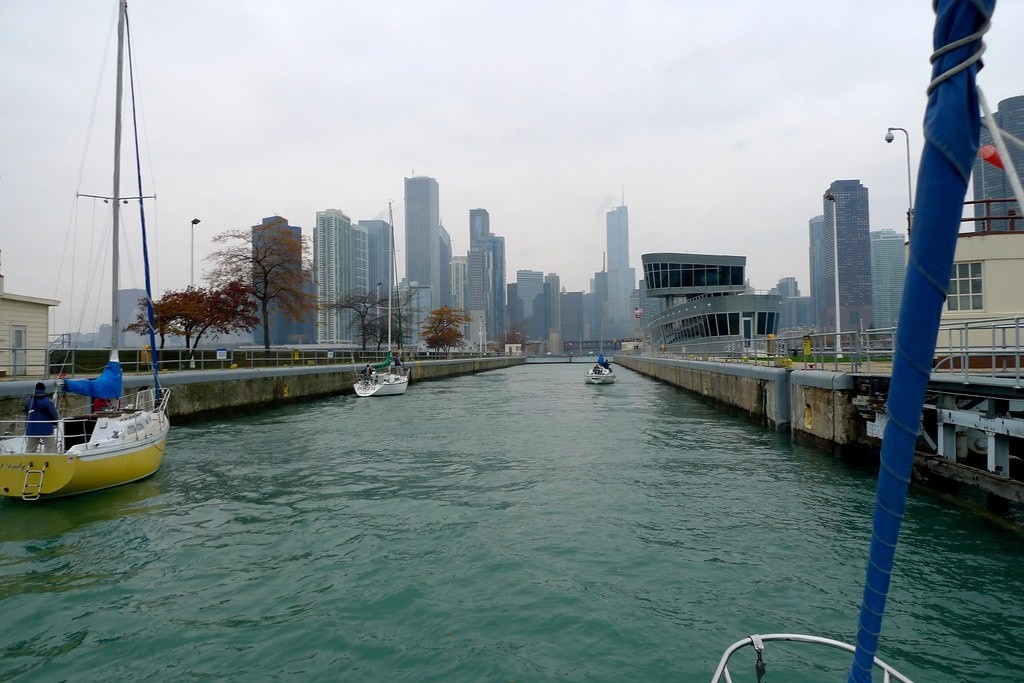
0;0;175;501
352;200;410;397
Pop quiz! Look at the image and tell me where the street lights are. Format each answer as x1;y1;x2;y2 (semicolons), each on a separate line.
825;193;845;358
186;218;202;290
374;280;384;344
885;127;912;231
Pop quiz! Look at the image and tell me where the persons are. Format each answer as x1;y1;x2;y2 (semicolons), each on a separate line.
364;364;374;384
602;359;611;370
23;382;58;453
597;352;605;365
593;366;601;374
395;355;404;376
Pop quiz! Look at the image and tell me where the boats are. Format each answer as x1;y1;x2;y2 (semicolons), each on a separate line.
584;362;616;384
